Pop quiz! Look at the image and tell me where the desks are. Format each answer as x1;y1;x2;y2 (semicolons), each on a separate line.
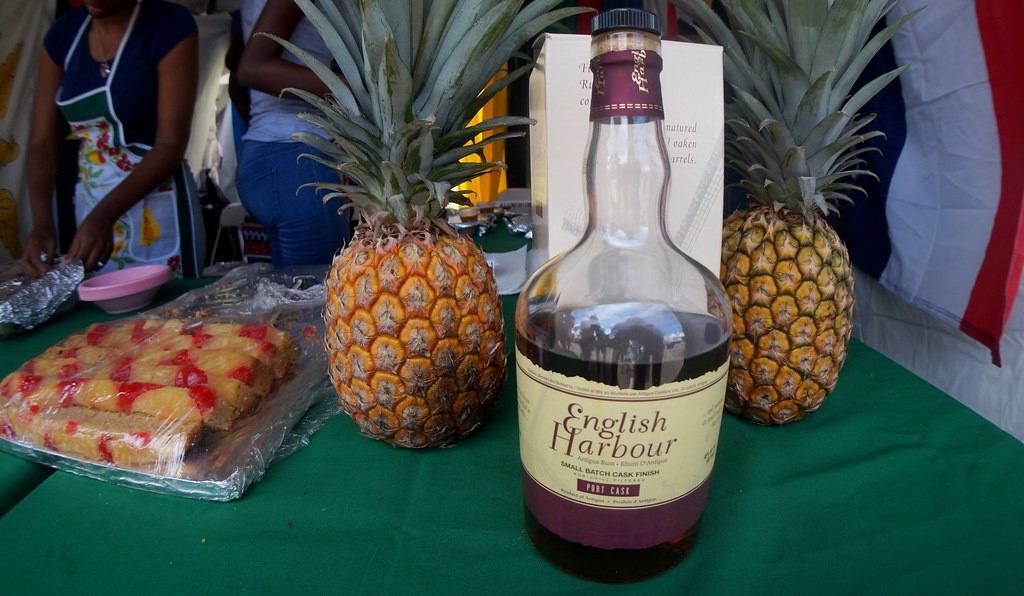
0;226;1024;596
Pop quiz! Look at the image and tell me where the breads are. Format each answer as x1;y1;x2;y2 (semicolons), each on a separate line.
0;319;302;464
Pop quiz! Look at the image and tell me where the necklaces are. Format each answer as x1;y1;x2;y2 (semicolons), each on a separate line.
93;17;115;78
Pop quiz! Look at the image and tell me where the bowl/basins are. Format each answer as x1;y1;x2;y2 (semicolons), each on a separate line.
77;264;172;315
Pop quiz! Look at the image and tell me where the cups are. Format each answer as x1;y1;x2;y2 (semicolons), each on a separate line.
458;205;481;223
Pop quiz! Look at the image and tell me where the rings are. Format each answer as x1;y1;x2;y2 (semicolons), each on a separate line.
98;261;103;266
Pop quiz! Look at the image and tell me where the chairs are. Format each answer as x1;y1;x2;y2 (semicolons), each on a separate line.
211;203;249;264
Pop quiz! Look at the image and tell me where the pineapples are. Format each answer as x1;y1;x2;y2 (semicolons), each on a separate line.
671;0;929;424
254;0;599;448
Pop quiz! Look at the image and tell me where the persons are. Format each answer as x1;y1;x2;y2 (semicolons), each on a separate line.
20;0;207;280
236;0;350;266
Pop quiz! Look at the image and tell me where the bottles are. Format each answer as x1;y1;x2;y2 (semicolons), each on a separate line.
514;7;733;583
488;206;514;234
